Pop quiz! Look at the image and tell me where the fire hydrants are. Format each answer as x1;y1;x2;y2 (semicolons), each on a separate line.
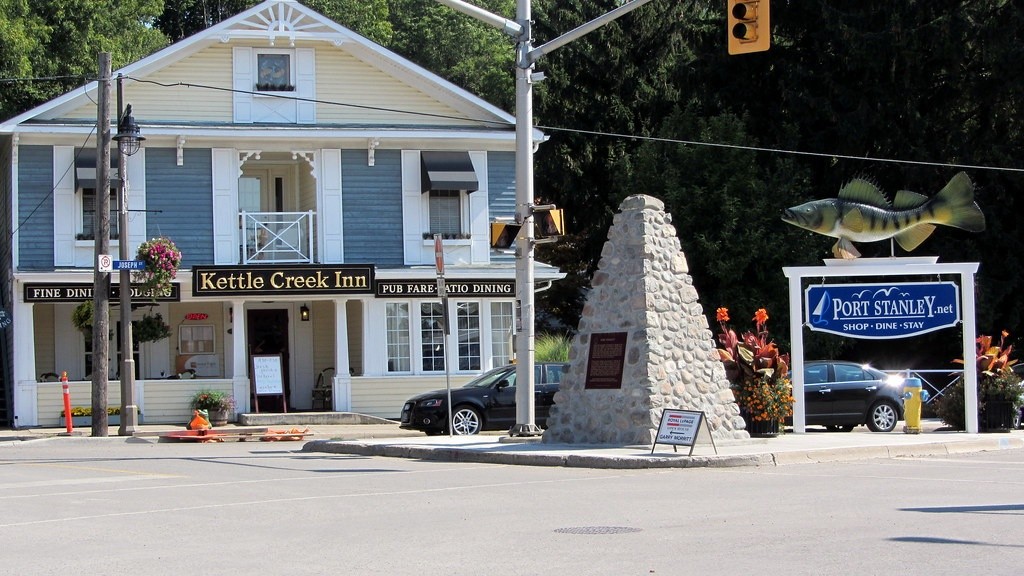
897;377;931;434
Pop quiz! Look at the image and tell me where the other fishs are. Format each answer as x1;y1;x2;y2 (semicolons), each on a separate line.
780;170;987;259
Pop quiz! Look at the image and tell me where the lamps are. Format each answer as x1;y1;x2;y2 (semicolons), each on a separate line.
300;303;310;321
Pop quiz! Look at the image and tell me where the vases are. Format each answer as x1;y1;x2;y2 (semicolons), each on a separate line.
208;410;228;427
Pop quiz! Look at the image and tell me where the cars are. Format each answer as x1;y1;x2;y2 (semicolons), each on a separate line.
779;358;906;432
399;360;566;436
1013;380;1024;430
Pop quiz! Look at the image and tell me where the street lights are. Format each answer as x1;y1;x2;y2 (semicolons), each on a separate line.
111;103;149;437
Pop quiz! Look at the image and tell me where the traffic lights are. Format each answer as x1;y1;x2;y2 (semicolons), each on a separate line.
490;222;521;249
727;0;770;55
533;207;565;239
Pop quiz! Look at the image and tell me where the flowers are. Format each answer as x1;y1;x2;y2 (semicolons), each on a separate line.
190;389;238;419
132;235;182;303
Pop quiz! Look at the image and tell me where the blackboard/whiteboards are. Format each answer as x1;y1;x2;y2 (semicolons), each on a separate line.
251;354;284;395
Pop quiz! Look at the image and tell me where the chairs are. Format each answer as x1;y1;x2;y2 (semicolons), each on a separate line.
311;367;335;411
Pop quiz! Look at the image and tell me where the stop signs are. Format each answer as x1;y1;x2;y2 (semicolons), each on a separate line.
433;233;444;276
